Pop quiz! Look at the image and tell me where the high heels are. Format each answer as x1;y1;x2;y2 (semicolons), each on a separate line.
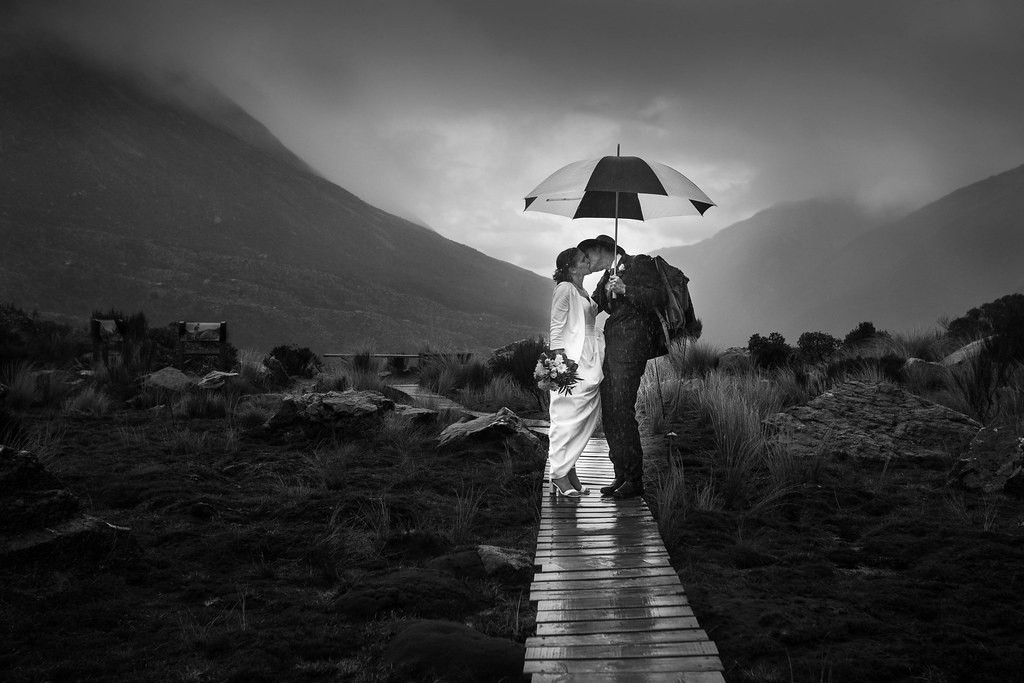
549;478;581;497
571;466;590;492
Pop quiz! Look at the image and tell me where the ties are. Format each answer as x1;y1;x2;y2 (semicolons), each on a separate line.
607;268;614;312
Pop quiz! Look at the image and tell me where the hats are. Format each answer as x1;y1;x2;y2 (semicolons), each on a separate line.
578;235;626;257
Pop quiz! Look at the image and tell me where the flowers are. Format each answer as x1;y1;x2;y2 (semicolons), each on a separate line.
534;350;585;396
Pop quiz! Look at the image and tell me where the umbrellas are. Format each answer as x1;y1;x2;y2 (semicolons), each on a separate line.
523;144;719;299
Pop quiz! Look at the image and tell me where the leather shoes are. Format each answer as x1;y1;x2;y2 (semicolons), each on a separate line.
600;477;625;495
613;479;644;498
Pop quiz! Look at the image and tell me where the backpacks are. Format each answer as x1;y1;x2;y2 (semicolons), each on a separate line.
626;253;697;348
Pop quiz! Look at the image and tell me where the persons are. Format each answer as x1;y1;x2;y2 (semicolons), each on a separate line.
577;234;670;496
548;246;604;498
